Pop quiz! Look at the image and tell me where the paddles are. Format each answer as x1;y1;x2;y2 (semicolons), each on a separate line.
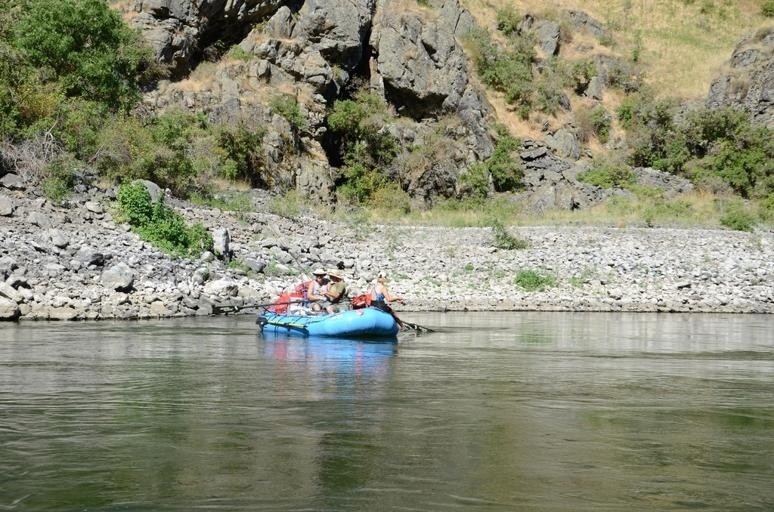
212;298;321;314
399;320;437;334
256;317;308;329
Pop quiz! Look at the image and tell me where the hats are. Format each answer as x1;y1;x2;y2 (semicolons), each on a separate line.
312;268;327;277
329;270;342;279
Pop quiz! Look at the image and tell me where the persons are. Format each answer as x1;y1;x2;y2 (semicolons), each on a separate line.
307;269;348;315
367;271;408;332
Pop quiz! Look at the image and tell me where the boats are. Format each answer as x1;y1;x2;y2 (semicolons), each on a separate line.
256;306;399;337
